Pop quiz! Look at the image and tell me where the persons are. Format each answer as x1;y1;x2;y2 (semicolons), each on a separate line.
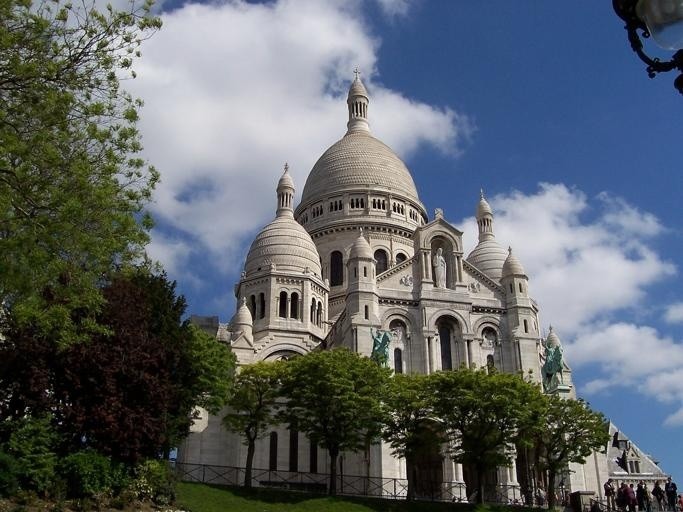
534;478;566;507
369;327;383;361
432;247;449;290
541;341;554;369
603;476;683;511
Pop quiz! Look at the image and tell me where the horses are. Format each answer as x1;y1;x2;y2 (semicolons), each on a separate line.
371;331;393;370
542;344;566;391
